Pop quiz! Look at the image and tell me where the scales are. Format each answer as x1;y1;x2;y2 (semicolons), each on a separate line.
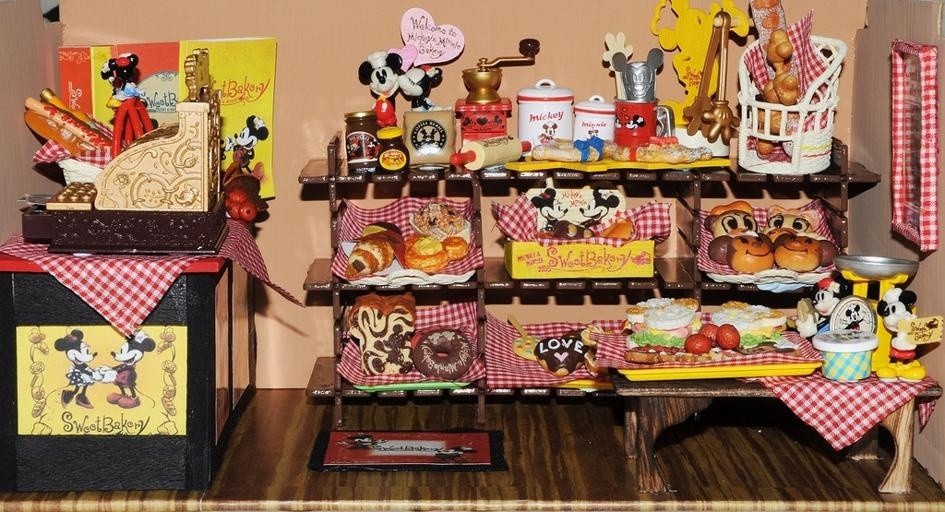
830;255;919;372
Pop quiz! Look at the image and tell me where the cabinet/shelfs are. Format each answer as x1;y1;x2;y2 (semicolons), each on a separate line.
299;135;877;429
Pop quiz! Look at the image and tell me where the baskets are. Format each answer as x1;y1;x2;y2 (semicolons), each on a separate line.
739;35;847;176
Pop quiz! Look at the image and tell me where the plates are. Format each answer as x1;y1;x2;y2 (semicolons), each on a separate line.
835;255;919;281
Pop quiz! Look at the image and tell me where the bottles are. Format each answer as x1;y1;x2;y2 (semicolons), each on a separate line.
518;80;573;156
573;95;615;144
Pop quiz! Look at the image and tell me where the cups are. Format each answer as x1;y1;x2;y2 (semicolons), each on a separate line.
615;99;674;146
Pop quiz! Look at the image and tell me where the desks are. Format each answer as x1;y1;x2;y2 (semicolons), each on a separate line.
603;355;939;496
2;252;258;496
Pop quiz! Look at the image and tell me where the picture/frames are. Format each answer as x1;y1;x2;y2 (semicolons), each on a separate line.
889;37;938;250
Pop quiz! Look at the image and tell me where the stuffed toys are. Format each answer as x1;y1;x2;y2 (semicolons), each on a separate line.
876;288;927;381
359;53;403;128
101;52;138;110
796;279;841;338
399;66;443;112
